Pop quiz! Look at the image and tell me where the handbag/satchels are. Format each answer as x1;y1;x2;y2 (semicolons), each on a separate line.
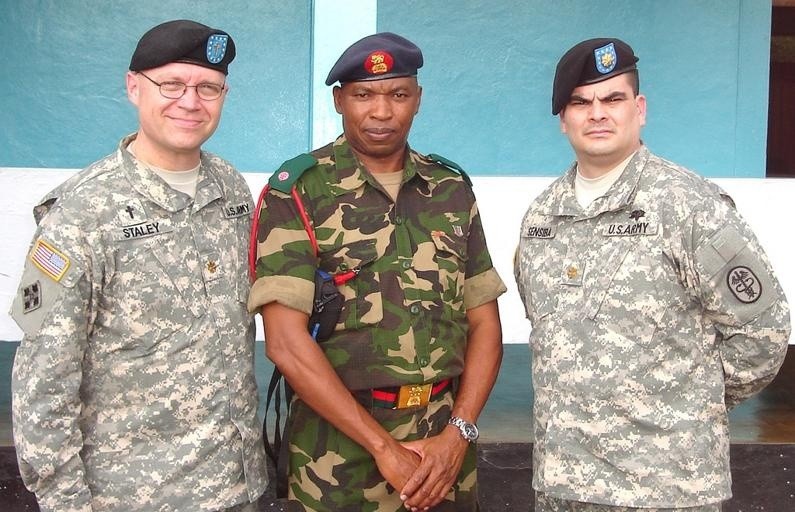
306;269;345;342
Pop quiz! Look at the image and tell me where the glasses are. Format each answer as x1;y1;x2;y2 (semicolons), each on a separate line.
139;71;226;101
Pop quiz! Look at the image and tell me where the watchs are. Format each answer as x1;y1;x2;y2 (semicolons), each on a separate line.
446;416;481;446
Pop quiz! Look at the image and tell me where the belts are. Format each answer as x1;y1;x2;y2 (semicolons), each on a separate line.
371;380;454;410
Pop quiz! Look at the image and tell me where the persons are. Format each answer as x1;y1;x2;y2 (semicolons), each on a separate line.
9;19;275;512
513;38;792;512
246;31;505;512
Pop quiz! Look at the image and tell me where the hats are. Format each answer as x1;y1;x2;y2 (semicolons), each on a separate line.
552;38;639;116
129;20;235;75
325;32;424;86
